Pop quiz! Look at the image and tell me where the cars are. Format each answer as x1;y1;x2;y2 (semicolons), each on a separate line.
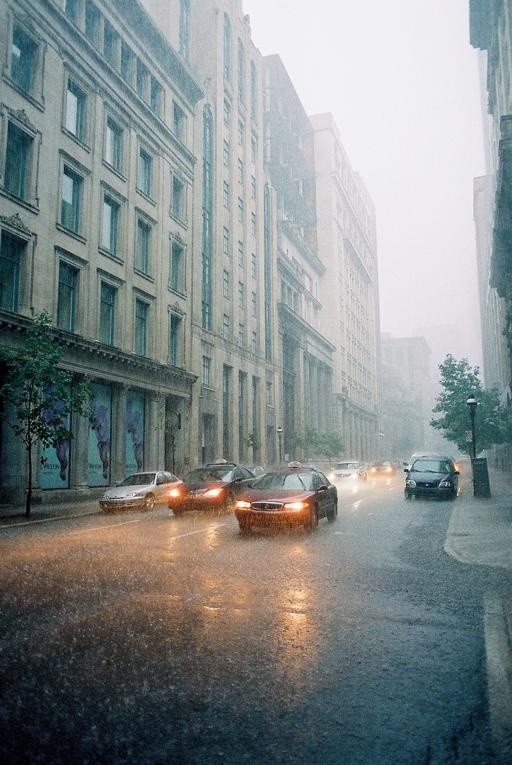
244;464;266;487
404;456;459;500
334;460;368;483
167;458;256;516
236;460;339;534
369;459;395;476
99;470;183;512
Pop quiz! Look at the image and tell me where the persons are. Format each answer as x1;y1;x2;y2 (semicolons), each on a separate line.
128;409;142;472
44;399;68;481
90;405;109;479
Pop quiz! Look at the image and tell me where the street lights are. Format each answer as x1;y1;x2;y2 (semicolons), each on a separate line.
276;426;283;463
467;393;478;458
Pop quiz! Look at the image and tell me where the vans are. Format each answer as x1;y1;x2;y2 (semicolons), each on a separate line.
404;452;442;487
